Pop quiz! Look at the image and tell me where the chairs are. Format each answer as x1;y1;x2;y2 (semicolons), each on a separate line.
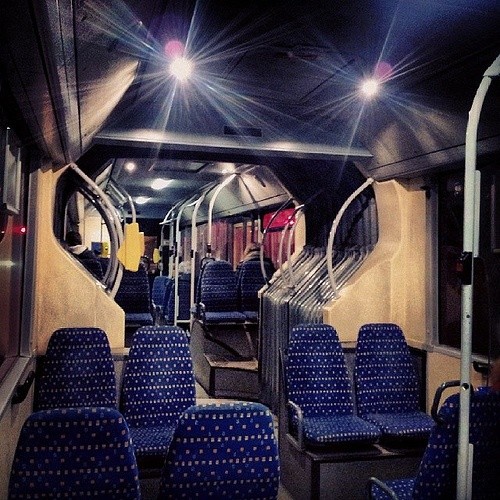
8;258;500;500
